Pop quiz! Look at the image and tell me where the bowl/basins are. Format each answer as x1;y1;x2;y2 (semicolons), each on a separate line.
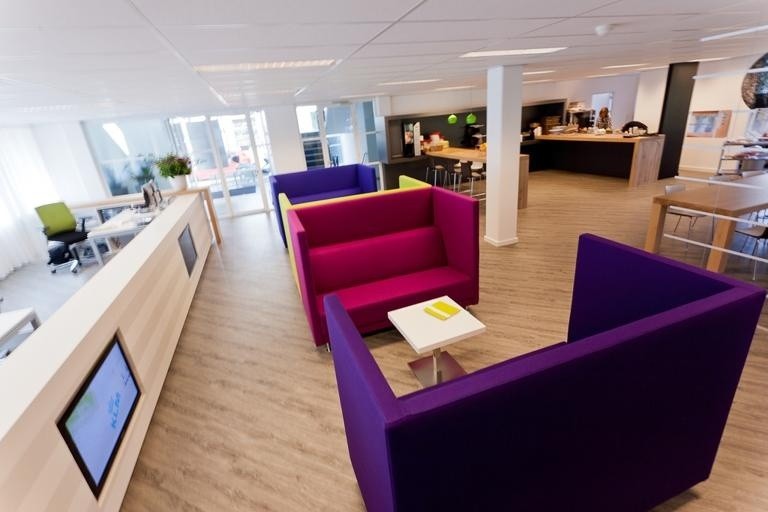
548;129;563;135
639;129;647;136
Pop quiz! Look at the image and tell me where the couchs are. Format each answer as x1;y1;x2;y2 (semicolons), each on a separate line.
269;163;377;248
278;174;433;303
322;233;768;512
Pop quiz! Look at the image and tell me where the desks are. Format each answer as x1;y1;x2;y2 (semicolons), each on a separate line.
0;308;41;348
87;207;159;269
644;173;768;273
426;147;530;209
535;131;666;187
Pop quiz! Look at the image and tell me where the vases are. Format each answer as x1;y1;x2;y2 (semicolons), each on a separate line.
167;175;187;192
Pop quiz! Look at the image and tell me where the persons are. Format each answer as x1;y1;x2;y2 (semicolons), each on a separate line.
596;107;611;130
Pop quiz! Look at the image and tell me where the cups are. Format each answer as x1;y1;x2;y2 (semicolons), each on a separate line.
625;126;638;136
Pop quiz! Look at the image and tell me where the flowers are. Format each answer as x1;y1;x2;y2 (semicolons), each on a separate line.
153;151;192;178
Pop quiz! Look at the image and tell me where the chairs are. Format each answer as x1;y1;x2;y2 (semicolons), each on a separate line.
35;202;90;274
734;225;768;281
285;185;480;354
665;184;707;256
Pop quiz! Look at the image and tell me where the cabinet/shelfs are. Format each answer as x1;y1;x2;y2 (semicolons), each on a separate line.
716;141;768;176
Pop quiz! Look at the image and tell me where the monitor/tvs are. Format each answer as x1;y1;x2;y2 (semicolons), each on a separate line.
142;182;159;208
57;332;142;499
148;177;163;202
179;223;198;277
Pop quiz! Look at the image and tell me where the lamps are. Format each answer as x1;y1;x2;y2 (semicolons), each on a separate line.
741;53;768;110
595;24;612;37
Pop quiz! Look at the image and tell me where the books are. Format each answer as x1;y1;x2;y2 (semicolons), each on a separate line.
423;300;461;321
